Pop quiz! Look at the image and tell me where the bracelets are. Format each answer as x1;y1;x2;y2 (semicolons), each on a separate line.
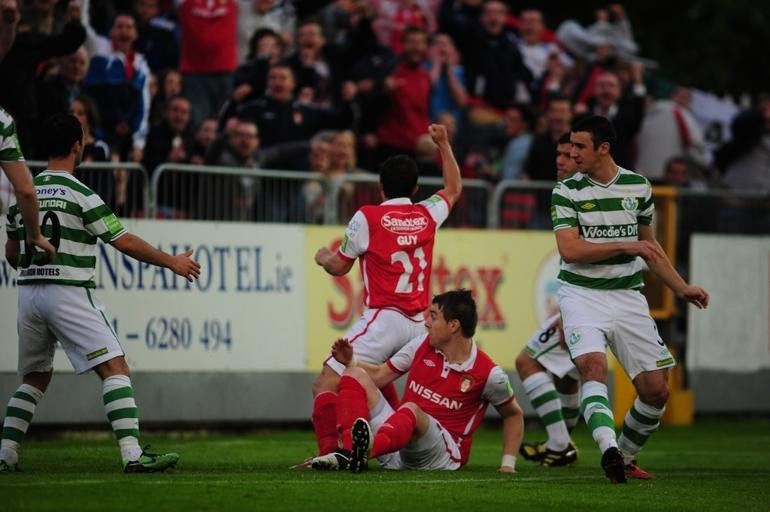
500;454;517;469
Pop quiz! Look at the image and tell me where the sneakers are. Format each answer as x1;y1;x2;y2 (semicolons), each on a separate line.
349;418;372;472
520;442;578;466
625;460;655;480
602;447;626;483
0;460;25;472
122;443;179;472
312;452;350;470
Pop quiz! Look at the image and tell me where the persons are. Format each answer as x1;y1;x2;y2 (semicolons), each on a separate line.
287;123;462;471
550;114;709;484
311;289;524;474
515;133;609;465
0;117;201;471
0;104;56;260
1;1;769;229
